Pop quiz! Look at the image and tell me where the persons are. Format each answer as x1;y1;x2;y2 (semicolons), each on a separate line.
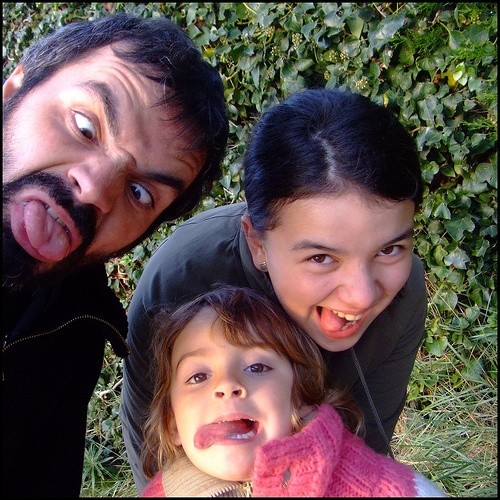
120;90;430;493
135;286;451;498
3;14;228;497
